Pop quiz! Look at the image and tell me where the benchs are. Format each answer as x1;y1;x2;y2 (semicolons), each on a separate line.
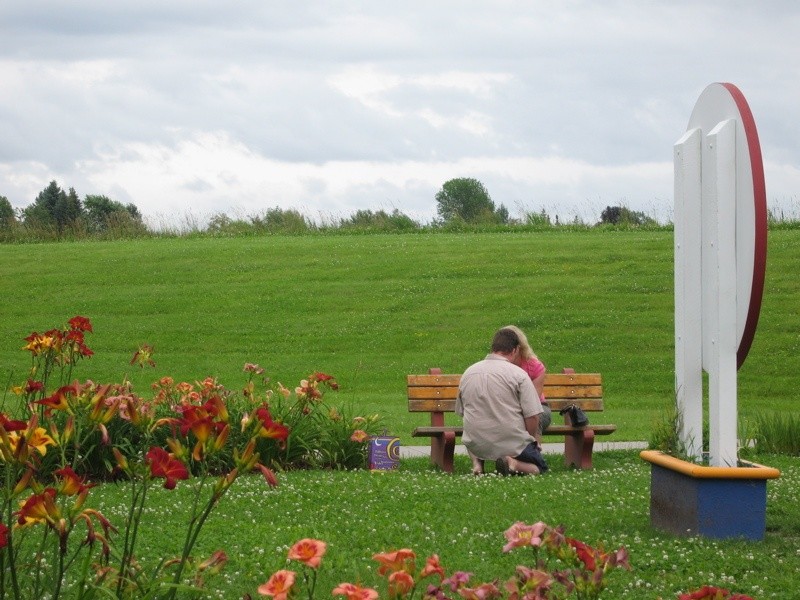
406;368;617;475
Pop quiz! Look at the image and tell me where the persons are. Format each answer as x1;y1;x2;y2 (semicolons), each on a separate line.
501;324;551;452
454;329;547;476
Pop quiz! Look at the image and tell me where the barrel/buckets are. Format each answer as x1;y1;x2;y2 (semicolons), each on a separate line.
368;428;400;470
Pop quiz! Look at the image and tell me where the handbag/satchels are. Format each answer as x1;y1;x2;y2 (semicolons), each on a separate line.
368;429;400;471
559;403;589;426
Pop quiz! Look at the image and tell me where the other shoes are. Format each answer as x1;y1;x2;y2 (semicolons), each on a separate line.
496;457;514;478
